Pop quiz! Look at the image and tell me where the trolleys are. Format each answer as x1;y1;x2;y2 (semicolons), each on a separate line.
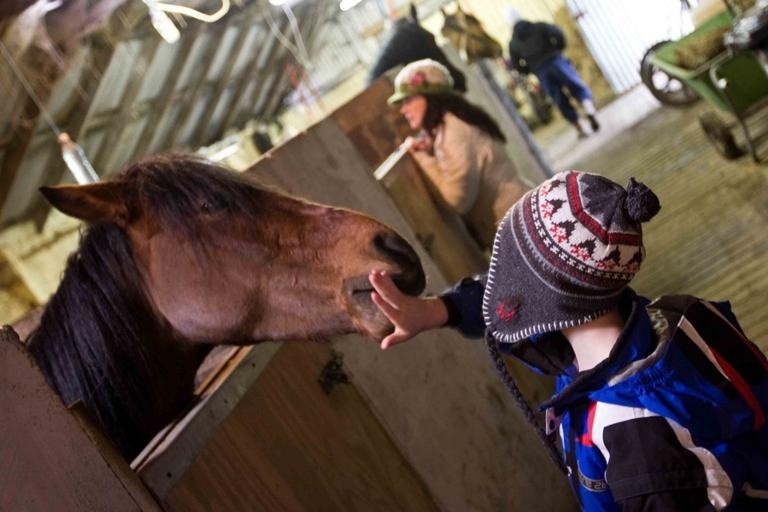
646;11;766;164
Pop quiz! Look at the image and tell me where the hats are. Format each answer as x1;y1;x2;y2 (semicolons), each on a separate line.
385;56;457;106
480;170;662;343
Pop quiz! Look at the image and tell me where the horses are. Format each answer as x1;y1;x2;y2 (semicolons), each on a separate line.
19;153;425;470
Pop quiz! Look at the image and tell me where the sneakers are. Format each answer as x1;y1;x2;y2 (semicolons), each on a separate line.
576;111;600;138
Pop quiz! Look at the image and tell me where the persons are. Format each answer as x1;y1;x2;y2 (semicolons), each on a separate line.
367;169;768;512
509;21;599;139
386;59;536;254
